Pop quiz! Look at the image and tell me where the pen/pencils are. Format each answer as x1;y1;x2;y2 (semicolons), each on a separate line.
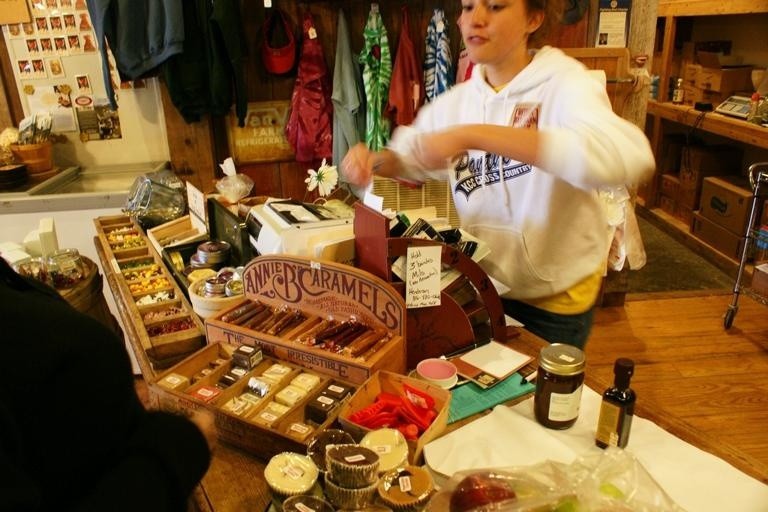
441;338;494;359
520;370;538;384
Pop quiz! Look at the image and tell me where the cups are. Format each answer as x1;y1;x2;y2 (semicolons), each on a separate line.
128;174;187;224
8;143;53;174
14;248;85;289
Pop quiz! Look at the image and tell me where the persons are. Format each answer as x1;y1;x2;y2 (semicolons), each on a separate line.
339;0;657;356
0;256;219;512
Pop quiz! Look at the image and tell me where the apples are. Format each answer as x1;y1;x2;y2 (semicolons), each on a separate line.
448;471;518;512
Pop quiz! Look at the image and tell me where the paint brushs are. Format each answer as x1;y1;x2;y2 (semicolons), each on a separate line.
17;114;52;145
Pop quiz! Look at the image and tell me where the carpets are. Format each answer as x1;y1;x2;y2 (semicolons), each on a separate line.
603;214;744;296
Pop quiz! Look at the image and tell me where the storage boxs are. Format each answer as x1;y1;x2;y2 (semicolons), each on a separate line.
338;368;453;465
691;211;755;261
677;58;755;111
657;142;739;223
695;170;763;237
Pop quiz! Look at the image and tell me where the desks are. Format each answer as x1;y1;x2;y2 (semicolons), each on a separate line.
91;232;768;512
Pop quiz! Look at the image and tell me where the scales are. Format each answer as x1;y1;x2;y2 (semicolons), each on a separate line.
715;92;768;122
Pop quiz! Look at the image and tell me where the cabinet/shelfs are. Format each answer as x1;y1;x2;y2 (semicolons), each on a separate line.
628;0;768;286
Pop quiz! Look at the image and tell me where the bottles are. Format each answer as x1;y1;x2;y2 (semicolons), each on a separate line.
590;356;636;448
672;78;685;106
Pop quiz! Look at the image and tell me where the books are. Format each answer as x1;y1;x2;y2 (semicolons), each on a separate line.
446;341;538;425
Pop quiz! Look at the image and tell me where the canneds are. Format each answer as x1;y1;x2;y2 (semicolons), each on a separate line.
534;342;587;431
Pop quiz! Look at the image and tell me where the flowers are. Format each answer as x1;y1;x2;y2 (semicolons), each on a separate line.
296;157;341;202
